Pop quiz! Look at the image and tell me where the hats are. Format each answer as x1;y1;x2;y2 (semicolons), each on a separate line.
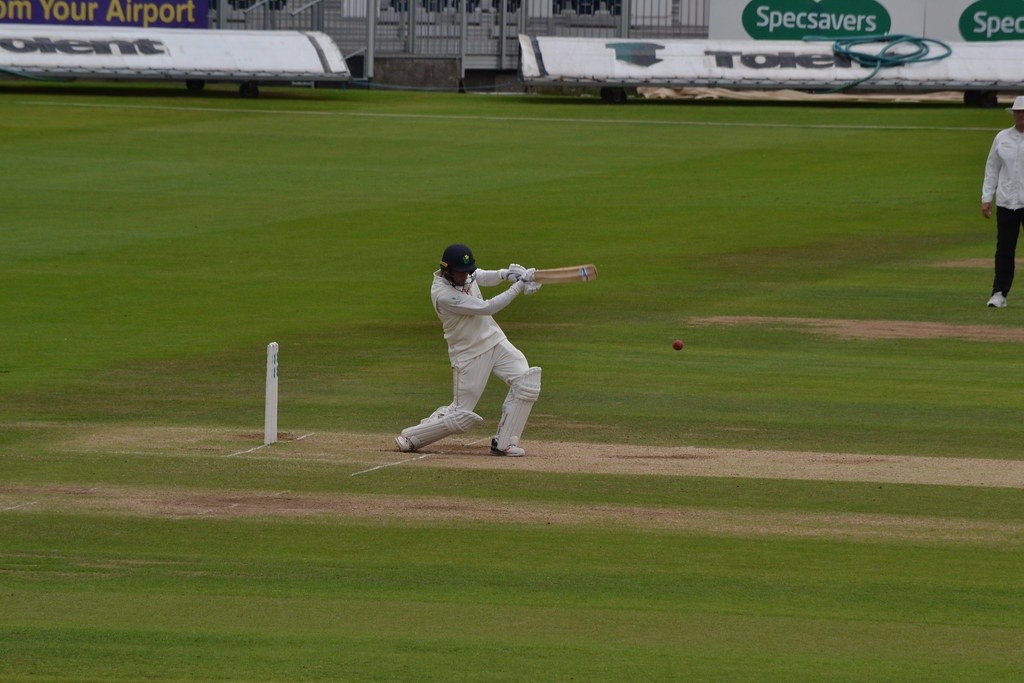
1005;96;1024;110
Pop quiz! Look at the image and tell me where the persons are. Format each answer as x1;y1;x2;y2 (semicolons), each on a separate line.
980;95;1024;307
396;245;543;457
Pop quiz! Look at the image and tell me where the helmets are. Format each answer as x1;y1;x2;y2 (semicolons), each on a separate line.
439;245;477;292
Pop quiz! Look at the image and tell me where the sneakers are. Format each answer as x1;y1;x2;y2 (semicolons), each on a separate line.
395;436;413;452
490;439;525;456
986;292;1007;307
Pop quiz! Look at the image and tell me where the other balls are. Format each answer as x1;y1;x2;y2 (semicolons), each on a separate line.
672;339;684;351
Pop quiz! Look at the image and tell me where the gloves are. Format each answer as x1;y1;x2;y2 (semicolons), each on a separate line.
500;264;526;283
516;268;542;296
